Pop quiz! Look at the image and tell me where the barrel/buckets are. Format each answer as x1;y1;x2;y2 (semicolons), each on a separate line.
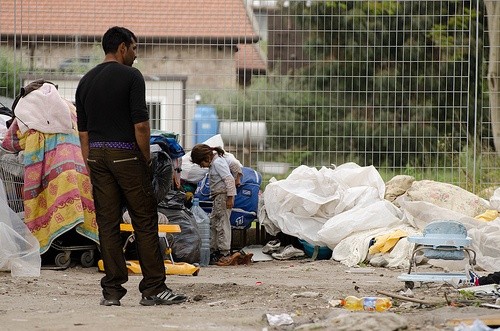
192;107;218;145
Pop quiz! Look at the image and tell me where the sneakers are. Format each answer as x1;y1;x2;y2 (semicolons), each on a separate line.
99;298;121;306
139;286;186;306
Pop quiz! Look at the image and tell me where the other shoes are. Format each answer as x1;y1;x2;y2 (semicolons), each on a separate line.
262;239;281;254
209;250;232;265
272;244;305;260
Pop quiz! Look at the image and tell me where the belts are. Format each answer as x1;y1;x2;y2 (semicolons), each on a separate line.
89;141;139;151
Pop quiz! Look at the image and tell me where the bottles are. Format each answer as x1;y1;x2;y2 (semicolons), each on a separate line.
341;296;392;312
190;198;211;267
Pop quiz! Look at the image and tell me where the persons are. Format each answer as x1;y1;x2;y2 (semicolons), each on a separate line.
190;143;244;264
74;26;189;307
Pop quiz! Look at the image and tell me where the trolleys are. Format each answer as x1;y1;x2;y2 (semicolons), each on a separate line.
0;159;98;268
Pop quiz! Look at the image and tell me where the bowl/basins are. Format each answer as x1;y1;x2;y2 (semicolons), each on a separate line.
258;161;291;175
